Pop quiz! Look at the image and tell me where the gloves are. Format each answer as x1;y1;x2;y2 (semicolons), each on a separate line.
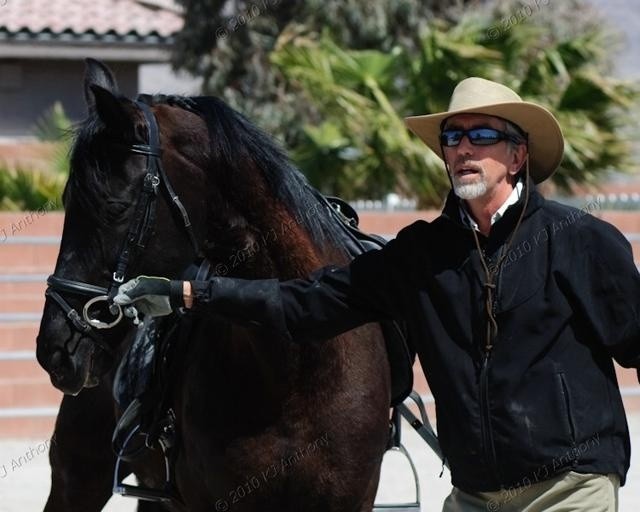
109;275;187;327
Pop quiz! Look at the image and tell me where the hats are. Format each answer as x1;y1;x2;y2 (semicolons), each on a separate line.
403;76;565;187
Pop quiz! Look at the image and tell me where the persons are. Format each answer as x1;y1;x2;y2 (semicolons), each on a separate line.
110;73;640;512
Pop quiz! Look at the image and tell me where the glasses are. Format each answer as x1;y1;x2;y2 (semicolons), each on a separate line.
437;127;519;148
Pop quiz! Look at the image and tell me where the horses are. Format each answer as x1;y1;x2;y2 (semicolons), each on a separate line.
35;58;392;512
